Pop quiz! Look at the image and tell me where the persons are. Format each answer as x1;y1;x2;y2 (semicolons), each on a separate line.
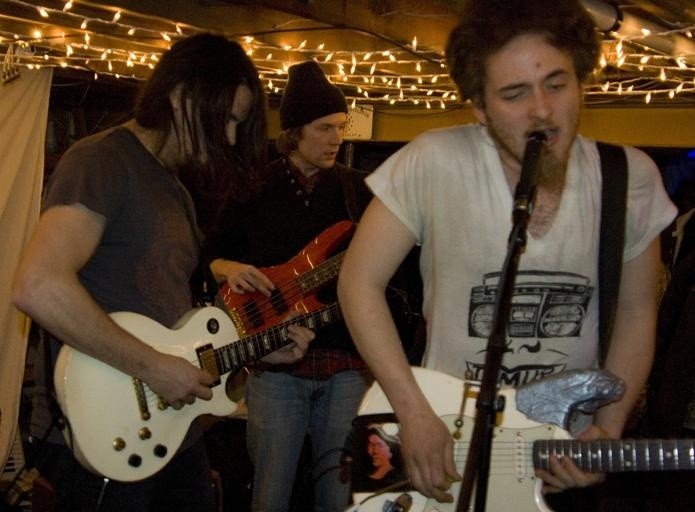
194;59;377;512
11;31;322;511
362;425;405;482
333;1;681;512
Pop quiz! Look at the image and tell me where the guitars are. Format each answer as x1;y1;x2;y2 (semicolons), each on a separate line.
216;221;357;340
347;365;694;511
51;284;422;483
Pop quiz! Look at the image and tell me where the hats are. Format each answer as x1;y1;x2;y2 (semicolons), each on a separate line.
279;62;347;131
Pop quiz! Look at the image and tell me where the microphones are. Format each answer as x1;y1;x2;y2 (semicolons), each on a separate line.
512;131;548;218
